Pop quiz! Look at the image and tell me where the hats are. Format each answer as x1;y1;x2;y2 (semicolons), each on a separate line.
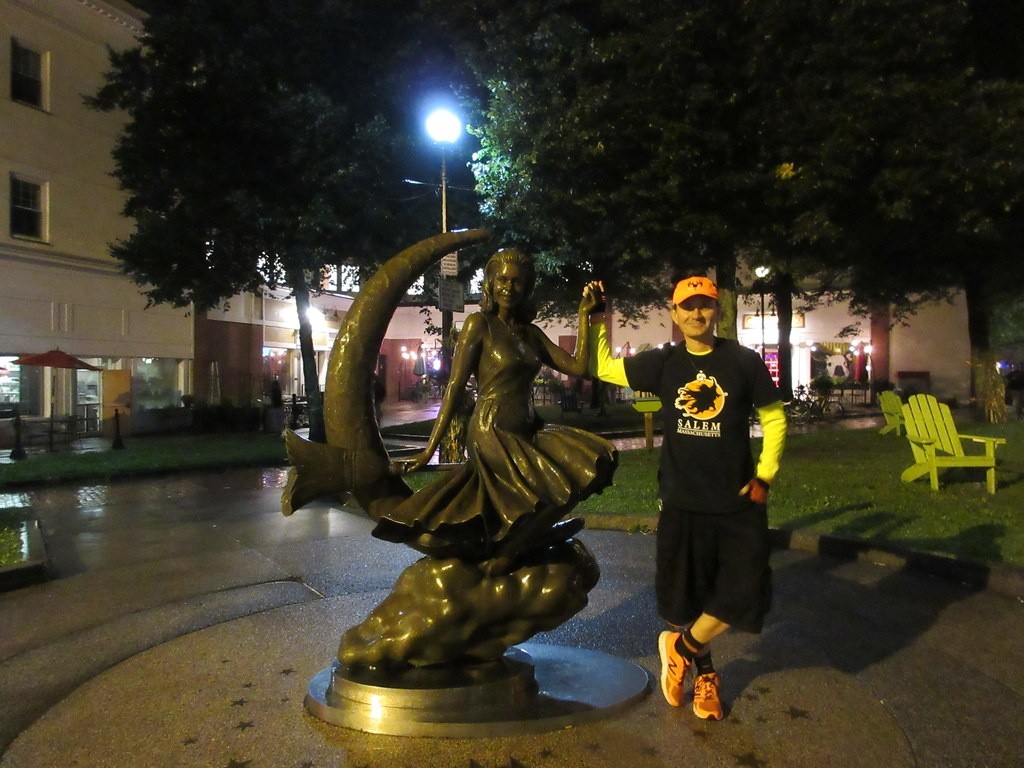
673;277;720;305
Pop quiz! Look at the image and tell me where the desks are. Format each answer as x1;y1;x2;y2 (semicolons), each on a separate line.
26;416;67;454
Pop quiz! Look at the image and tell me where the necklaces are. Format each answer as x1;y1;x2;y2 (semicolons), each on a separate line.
686;348;715;382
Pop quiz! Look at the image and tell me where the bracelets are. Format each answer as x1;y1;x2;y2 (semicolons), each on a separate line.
590;316;606;321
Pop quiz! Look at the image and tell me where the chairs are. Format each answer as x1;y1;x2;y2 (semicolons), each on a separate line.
20;415;84;451
900;394;1006;494
880;390;905;436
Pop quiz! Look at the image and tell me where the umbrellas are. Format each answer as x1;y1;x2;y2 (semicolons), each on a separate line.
10;351;102;419
854;343;866;385
413;346;425;380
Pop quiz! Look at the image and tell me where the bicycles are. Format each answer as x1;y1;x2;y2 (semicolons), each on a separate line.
786;388;844;423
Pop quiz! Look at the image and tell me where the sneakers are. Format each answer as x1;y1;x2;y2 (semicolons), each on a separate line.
658;630;693;707
692;672;723;721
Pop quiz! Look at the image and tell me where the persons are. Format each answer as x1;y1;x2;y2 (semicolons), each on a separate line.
374;376;386;425
582;276;787;721
371;248;617;551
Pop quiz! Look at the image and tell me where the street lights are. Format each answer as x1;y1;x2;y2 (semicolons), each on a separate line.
398;346;410;403
865;342;876;405
426;105;462;466
850;338;858;406
616;345;621;400
756;266;769;364
